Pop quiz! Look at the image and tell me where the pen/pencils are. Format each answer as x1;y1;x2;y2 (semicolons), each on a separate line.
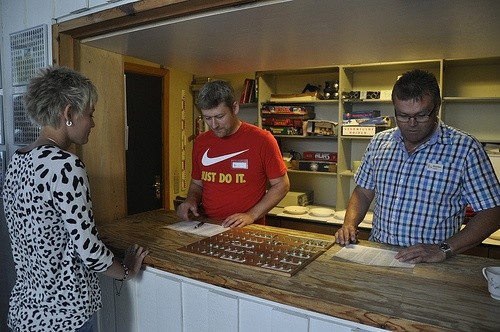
348;230;359;241
195;221;204;228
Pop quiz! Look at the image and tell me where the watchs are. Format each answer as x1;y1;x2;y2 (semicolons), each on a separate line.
439;242;452;259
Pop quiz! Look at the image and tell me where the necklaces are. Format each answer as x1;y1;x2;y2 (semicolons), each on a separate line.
48;136;60;147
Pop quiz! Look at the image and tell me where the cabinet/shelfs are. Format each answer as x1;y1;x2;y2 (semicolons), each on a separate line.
339;59;441;213
441;57;500;168
191;72;259;141
256;66;339;212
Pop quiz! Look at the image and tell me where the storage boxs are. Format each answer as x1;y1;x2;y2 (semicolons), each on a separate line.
261;103;377;207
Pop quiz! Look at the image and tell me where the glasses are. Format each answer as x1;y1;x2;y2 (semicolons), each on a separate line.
395;104;437;123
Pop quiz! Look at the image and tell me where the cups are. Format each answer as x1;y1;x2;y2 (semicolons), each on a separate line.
482;266;500;300
353;160;361;171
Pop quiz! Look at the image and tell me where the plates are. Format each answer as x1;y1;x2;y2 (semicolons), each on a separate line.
333;210;347;219
284;206;309;215
363;212;373;224
309;208;334;217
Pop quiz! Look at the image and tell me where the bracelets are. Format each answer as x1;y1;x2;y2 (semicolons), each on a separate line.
114;264;128;296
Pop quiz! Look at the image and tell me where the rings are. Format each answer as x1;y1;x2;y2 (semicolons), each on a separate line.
240;218;243;221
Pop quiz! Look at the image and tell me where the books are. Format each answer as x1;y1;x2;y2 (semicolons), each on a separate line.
342;110;395;137
239;78;258;103
261;103;338;136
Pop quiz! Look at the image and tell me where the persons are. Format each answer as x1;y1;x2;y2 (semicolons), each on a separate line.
2;65;150;332
334;69;500;264
176;79;290;228
